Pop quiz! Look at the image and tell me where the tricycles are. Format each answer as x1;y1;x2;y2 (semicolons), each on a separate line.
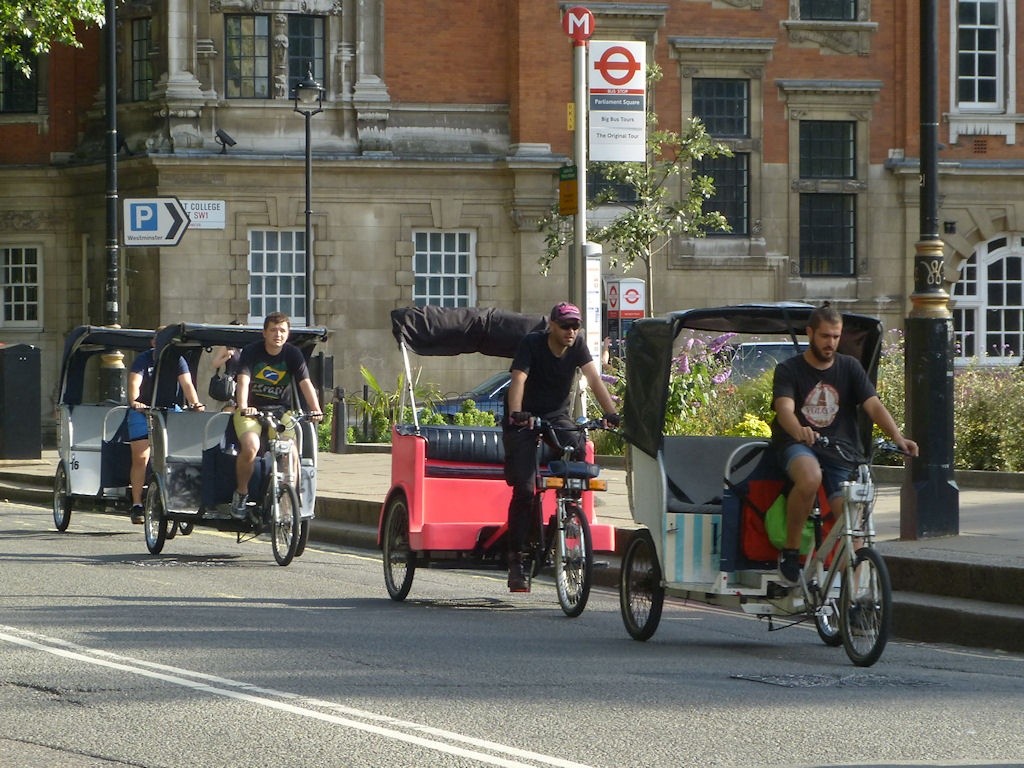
52;323;196;541
142;321;329;567
616;301;920;668
376;305;625;619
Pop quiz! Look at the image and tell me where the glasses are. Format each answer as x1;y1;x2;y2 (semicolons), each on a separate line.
559;322;580;331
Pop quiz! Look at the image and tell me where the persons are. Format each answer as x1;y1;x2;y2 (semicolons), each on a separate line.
231;312;323;519
503;301;619;589
771;305;919;597
212;320;244;412
127;325;205;524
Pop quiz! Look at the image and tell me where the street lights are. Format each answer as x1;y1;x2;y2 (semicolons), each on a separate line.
292;60;328;328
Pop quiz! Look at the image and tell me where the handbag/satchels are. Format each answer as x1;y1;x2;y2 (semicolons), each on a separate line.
209;375;236;402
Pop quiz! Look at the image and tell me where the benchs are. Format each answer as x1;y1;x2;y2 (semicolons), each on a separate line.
663;436;767;514
153;410;319;466
397;425;551;479
55;405;127;451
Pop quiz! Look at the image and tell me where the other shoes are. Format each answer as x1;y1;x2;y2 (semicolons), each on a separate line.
230;490;250;519
508;565;527;590
130;505;146;525
777;549;802;586
550;514;580;536
850;605;876;637
280;519;293;533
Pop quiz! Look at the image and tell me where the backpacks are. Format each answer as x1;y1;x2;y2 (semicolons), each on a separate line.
725;441;819;554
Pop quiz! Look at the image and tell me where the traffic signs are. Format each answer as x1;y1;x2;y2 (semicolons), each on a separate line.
123;196;192;247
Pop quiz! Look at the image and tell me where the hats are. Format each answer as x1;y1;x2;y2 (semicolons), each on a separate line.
551;301;583;324
